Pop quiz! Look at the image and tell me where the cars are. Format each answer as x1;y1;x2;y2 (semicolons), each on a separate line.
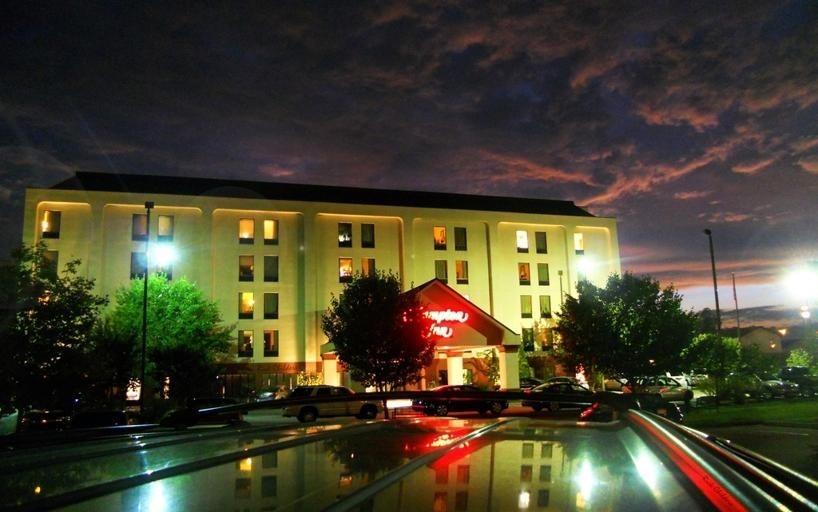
19;408;147;436
521;373;798;412
281;384;383;422
161;397;249;429
411;384;509;416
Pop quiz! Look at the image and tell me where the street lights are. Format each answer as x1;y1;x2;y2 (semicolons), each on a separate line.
703;227;721;335
139;201;156;429
732;272;742;345
